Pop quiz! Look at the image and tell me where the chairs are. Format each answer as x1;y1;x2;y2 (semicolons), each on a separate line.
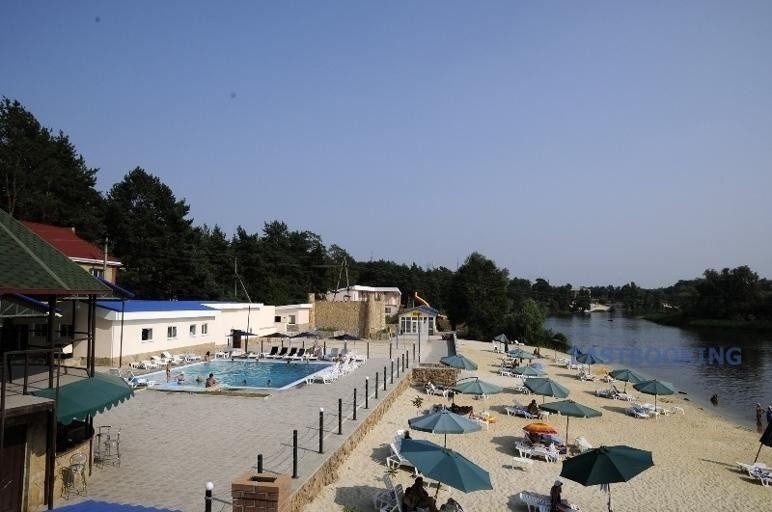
550;349;672;417
371;429;436;511
515;436;560;464
111;351;202;389
60;423;123;499
426;379;452;398
735;458;772;489
503;403;551;421
215;346;351;364
519;490;568;512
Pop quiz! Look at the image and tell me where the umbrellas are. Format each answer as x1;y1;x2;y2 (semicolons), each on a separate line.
290;330;320;348
329;333;360;352
450;375;503;397
559;445;655;512
407;407;483;450
758;416;771;448
511;363;547;378
537;398;602;445
225;330;258;338
508;348;539;360
494;332;507;348
565;343;582;363
574;352;604;375
440;353;479;372
523;376;570;403
608;367;646;392
399;436;494;495
261;332;289;343
633;377;675;411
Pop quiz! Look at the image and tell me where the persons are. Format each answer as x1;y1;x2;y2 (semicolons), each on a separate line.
207;372;215;385
427;381;435;393
528;431;551;443
412;475;438;511
451;402;473;412
550;479;575;511
511;358;517;368
204;350;211;363
765;405;772;424
756;403;764;424
537;345;540;356
534;348;537;354
503;339;508;352
402;486;419;512
197;375;204;382
177;371;185;384
166;359;172;383
527;398;538;415
335;353;344;372
